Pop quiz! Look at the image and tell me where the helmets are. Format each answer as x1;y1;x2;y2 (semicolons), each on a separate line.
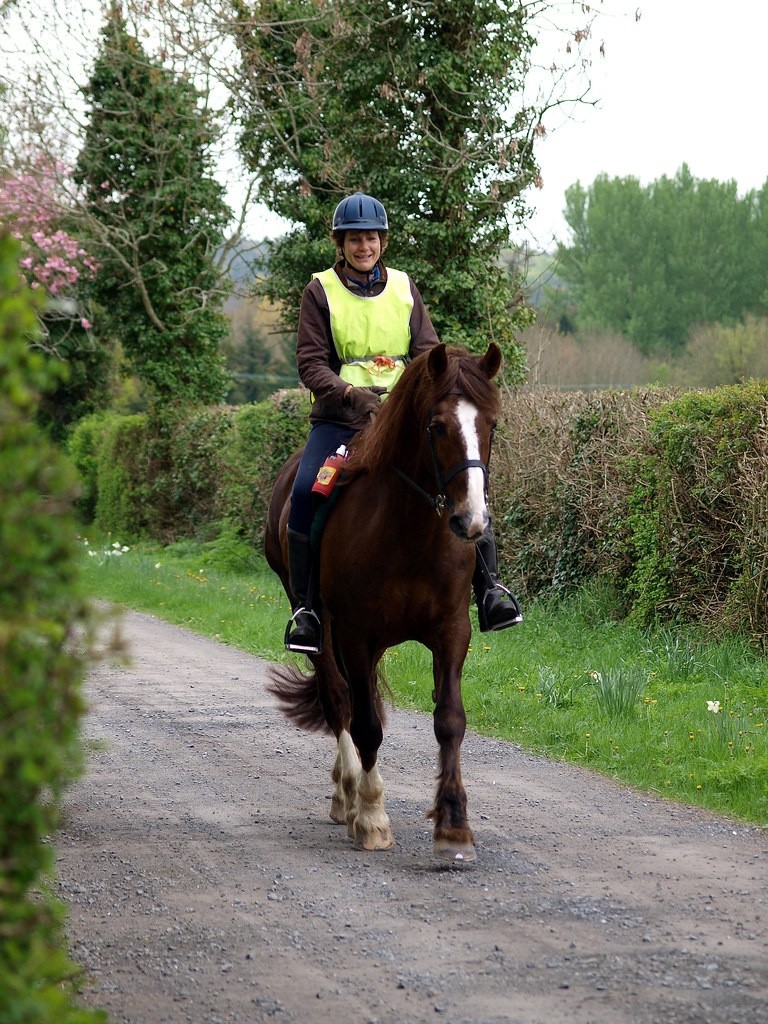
331;191;389;231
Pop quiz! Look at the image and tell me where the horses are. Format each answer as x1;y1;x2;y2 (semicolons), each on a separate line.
262;338;511;866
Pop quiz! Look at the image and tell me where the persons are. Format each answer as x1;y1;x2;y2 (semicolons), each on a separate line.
284;191;522;652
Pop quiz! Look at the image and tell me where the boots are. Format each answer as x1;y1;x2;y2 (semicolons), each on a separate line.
286;523;317;642
467;517;518;633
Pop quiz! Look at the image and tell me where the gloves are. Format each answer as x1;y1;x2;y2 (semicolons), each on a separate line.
344;385;387;418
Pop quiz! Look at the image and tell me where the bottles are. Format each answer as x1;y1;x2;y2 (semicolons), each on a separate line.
311;445;346;498
